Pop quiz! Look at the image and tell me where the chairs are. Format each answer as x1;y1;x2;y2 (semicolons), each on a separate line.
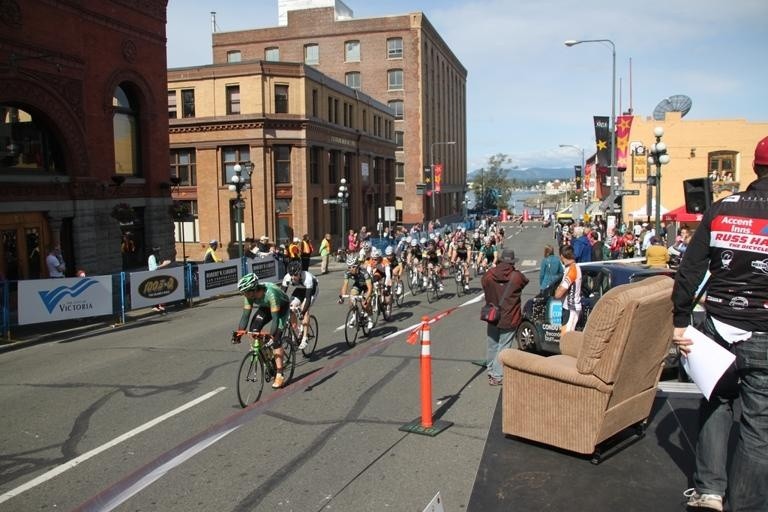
499;275;675;465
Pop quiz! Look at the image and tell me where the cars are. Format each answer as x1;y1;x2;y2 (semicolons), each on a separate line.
516;263;686;377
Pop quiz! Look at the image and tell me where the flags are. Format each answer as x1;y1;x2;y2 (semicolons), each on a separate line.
593;116;611;167
616;116;632;168
424;166;432;190
434;165;442;192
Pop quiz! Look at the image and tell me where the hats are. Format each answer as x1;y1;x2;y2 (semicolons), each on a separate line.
754;135;768;166
260;235;300;248
498;250;521;264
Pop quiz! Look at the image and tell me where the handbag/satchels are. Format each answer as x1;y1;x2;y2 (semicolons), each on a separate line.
477;304;501;322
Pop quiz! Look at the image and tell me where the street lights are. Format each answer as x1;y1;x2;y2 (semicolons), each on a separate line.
335;176;352;255
647;126;668;246
243;160;257;245
230;157;247;256
561;35;620;229
429;139;459;227
559;143;587;205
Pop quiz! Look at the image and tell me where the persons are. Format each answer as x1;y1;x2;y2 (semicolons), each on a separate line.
538;190;701;360
46;243;66;279
204;212;508;387
479;250;530;384
669;136;768;510
147;246;172;313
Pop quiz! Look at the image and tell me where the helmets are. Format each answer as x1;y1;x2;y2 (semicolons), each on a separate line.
237;272;259;293
287;261;303;276
338;215;504;268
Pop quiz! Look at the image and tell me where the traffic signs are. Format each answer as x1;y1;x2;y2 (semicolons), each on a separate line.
615;189;639;196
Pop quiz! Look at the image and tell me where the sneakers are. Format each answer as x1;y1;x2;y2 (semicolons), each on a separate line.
270;372;286;389
297;336;309;349
685;492;725;511
487;376;502;387
342;269;471;333
